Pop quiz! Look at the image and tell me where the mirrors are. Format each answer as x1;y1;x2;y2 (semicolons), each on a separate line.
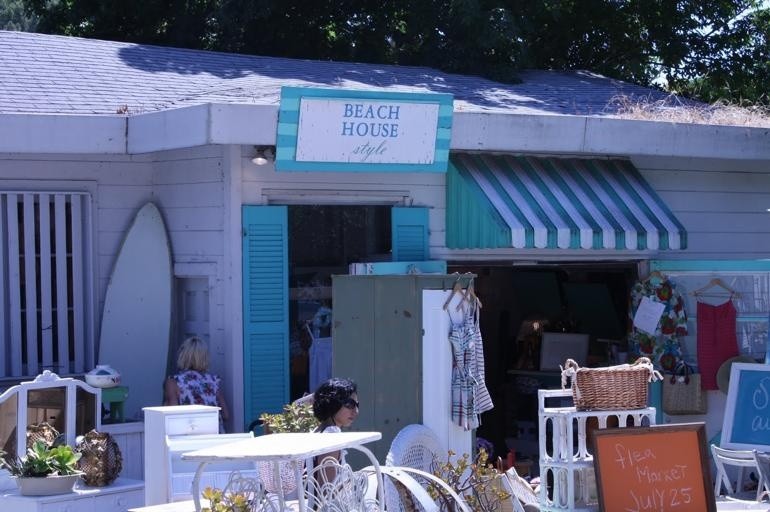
72;380;101;437
23;371;73;452
2;386;25;489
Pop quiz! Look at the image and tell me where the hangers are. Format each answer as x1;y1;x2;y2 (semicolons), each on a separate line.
690;273;743;301
456;273;482;312
443;271;471;309
642;263;666;290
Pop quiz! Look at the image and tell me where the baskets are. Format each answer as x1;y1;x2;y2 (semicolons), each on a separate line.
564;356;652;411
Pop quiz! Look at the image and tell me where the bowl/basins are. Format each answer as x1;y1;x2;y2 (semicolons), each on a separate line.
84;364;121;388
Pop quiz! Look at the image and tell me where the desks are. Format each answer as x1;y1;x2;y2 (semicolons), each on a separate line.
180;432;382;512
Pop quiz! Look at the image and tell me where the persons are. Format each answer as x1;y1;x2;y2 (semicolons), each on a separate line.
304;379;359;511
164;337;229;434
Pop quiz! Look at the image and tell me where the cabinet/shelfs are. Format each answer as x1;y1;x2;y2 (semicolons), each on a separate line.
143;405;220;505
538;388;655;512
164;432;261;502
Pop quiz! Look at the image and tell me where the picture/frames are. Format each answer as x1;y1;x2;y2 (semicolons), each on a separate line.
591;421;718;512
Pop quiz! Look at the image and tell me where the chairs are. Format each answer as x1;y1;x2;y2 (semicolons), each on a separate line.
704;445;770;509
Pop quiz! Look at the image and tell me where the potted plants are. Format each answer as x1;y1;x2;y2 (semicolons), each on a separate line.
10;440;85;498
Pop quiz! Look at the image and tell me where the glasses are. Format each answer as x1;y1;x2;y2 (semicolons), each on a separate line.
344;397;360;409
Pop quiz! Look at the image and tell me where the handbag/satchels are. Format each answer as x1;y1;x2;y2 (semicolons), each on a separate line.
664;362;702;412
666;365;707;415
